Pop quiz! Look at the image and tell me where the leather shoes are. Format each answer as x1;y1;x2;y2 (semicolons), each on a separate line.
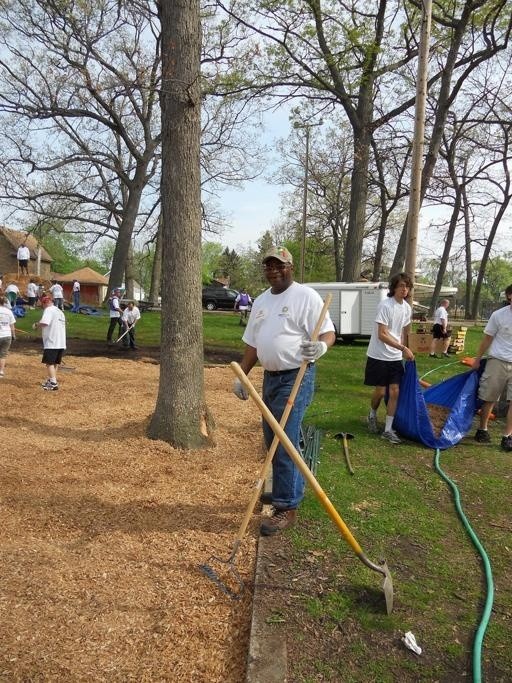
261;492;274;505
260;508;298;536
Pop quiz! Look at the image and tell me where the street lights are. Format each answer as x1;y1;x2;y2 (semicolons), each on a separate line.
292;115;325;282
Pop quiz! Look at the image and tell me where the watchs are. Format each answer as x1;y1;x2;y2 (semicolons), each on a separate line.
430;298;452;357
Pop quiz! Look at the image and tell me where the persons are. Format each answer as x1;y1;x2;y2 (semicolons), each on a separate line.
0;293;16;377
363;272;416;444
232;245;336;535
107;287;123;345
118;301;142;351
471;283;512;451
31;296;66;389
16;243;30;275
0;275;81;312
234;287;253;326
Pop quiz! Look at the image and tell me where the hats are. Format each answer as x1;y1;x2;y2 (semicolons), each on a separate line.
262;246;293;265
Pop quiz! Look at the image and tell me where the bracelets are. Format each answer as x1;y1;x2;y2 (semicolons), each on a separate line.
401;346;407;351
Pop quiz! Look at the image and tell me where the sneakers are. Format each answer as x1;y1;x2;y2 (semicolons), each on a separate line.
381;431;402;444
441;353;450;359
501;437;512;451
429;353;438;358
368;414;379;434
0;371;5;378
41;379;59;390
475;430;490;442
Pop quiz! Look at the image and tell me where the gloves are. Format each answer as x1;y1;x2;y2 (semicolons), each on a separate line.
300;340;327;363
232;377;249;400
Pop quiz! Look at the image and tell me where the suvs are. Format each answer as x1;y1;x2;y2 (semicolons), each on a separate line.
200;287;255;310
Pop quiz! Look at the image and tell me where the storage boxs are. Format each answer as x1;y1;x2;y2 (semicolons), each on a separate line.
407;332;433;353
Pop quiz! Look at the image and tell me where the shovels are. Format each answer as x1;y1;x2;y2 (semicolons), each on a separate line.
334;432;355;475
230;361;394;616
107;325;133;346
